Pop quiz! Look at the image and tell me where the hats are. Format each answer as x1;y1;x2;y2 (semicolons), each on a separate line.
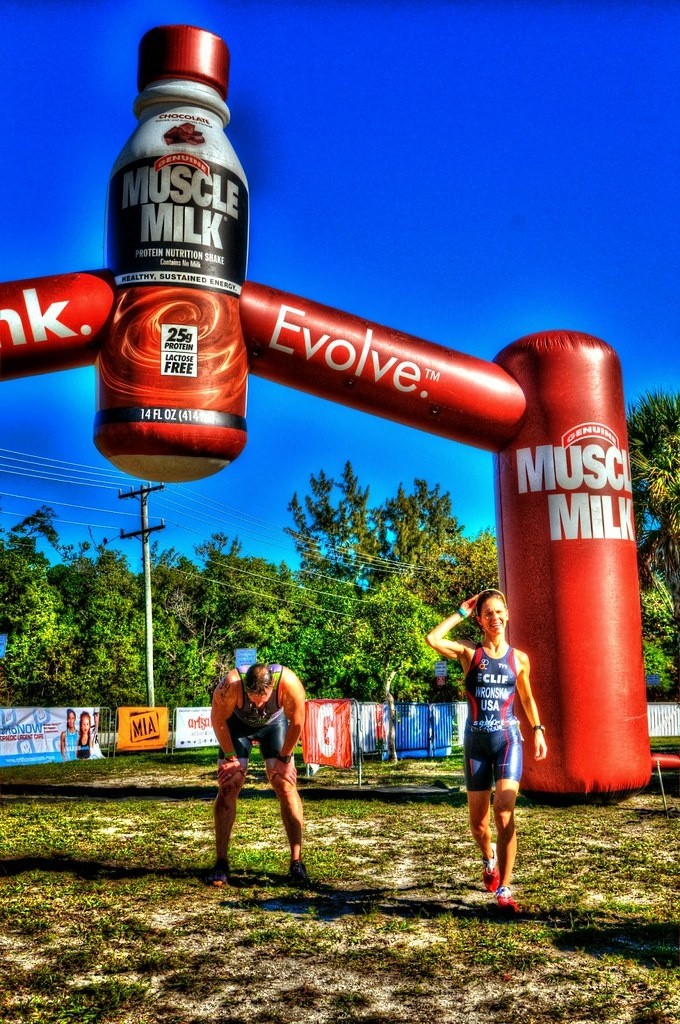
93;707;99;716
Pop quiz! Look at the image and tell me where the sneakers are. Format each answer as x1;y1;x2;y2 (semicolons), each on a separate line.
494;885;521;919
482;841;501;893
205;859;231;886
290;861;312;889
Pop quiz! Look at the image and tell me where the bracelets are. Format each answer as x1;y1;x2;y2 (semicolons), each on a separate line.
458;608;466;617
533;725;545;734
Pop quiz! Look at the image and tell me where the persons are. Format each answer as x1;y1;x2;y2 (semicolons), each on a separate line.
425;589;547;898
89;708;98;748
76;712;92;759
208;663;315;888
60;709;78;761
